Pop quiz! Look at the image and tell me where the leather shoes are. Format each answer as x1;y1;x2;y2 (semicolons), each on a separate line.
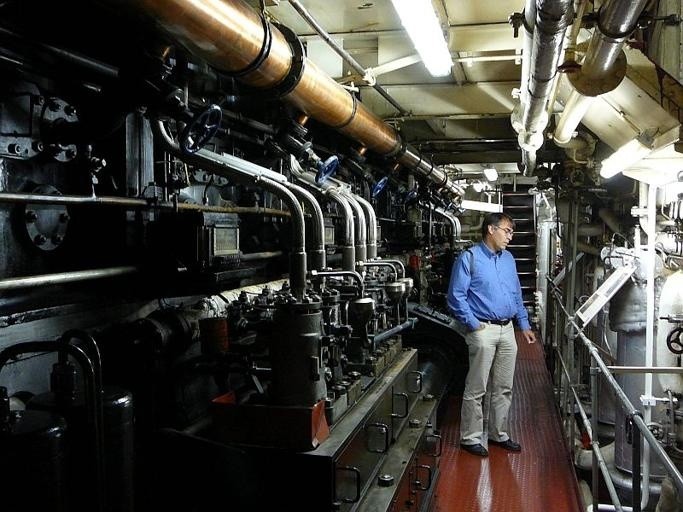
487;437;523;453
460;443;490;458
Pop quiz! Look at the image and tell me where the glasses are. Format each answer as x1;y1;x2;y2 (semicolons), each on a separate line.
493;224;515;237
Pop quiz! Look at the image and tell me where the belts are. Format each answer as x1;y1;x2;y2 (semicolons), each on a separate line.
477;317;514;326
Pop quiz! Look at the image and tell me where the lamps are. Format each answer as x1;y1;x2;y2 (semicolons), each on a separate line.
482;164;499;182
597;127;661;180
389;0;454;78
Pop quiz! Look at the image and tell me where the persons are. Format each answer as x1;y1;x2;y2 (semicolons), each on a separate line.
447;212;537;457
